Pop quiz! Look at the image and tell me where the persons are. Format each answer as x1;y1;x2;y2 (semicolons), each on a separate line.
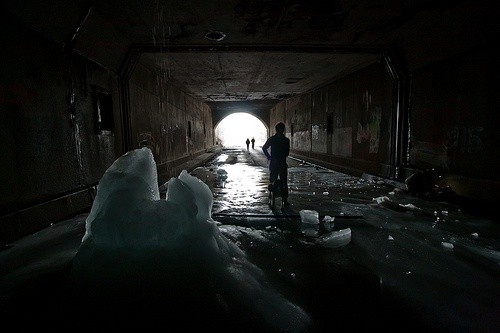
246;138;250;150
263;122;291;207
251;137;255;149
